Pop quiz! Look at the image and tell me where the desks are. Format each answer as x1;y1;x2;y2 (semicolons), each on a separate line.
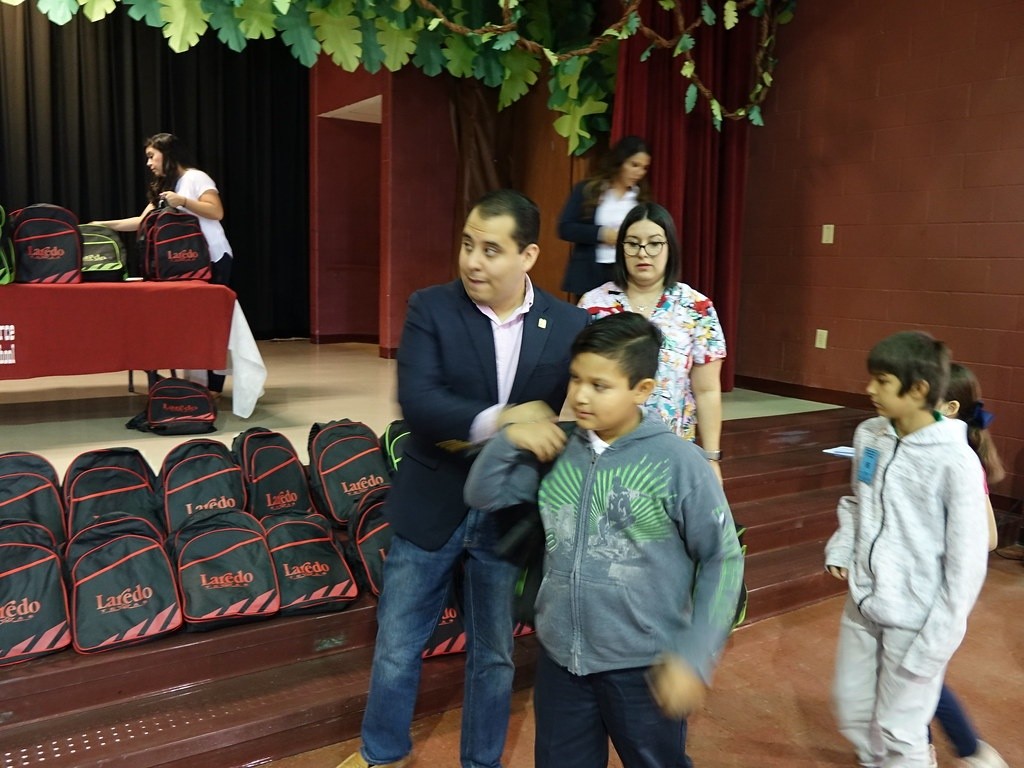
0;279;236;392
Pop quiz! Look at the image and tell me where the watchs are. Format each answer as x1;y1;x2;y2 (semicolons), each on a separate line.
706;450;723;461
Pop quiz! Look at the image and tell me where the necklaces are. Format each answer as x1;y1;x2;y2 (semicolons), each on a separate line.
637;306;648;312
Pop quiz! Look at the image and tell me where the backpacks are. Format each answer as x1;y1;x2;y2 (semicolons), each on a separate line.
0;420;534;661
136;197;212;281
9;204;82;284
79;223;128;282
126;378;218;435
695;520;748;634
0;205;14;285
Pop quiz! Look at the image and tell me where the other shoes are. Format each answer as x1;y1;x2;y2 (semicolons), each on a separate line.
953;738;1008;767
928;744;938;768
336;750;414;768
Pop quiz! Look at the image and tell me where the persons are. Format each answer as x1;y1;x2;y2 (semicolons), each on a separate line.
336;195;592;768
558;135;653;302
822;332;1005;768
85;133;234;398
463;310;745;767
572;201;726;489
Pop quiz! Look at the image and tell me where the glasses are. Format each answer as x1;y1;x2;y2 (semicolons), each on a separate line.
620;241;668;256
